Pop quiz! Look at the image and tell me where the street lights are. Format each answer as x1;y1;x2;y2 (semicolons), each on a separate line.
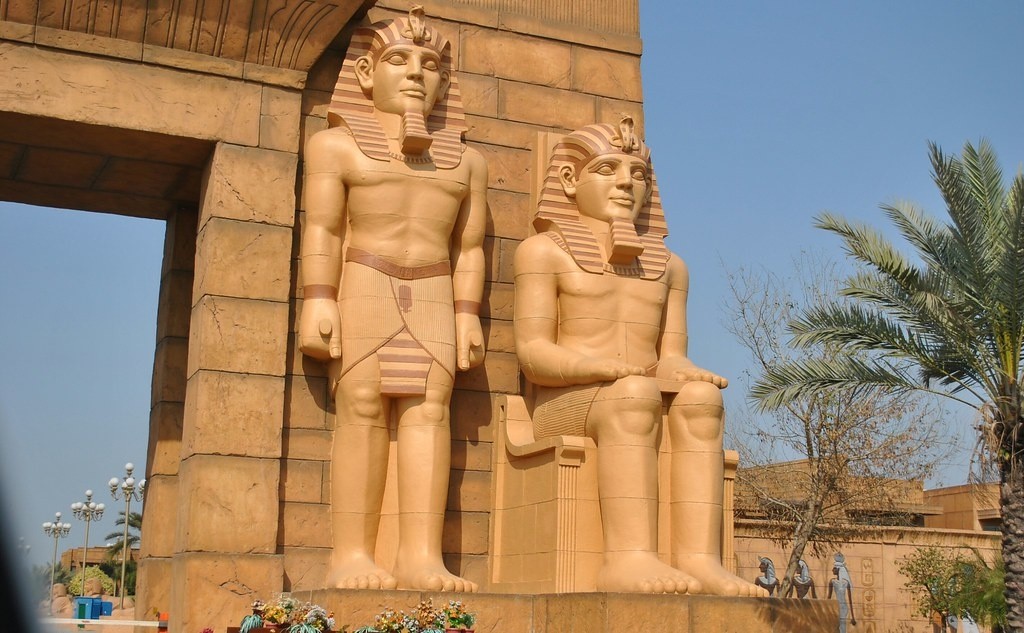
71;489;106;597
107;462;146;609
42;512;72;615
107;536;120;598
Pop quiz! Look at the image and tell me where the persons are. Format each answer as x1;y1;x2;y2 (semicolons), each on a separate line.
512;114;769;596
49;577;102;618
297;6;487;593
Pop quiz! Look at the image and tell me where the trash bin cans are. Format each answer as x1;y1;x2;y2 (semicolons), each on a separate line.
101;602;113;616
73;595;101;618
